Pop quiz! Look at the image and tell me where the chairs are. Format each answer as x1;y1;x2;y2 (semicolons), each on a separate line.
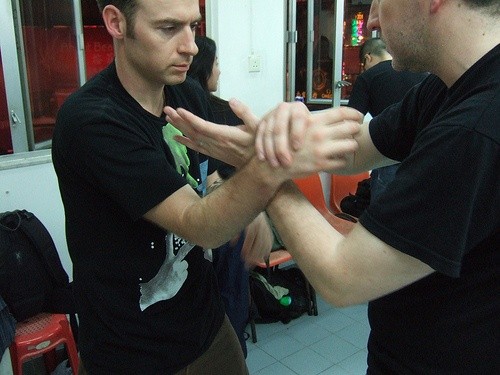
329;172;370;221
292;174;356;316
249;250;312;344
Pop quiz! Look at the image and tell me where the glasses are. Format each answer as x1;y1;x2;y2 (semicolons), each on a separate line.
362;53;370;70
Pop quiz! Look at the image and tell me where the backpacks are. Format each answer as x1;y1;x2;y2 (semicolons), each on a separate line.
0;209;78;322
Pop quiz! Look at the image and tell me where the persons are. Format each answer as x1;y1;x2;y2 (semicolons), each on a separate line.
52;0;429;375
164;0;500;375
312;35;334;96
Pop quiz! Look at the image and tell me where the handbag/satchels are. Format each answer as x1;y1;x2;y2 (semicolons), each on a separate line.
248;265;308;323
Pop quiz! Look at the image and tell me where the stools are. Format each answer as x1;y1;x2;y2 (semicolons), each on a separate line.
8;312;79;375
